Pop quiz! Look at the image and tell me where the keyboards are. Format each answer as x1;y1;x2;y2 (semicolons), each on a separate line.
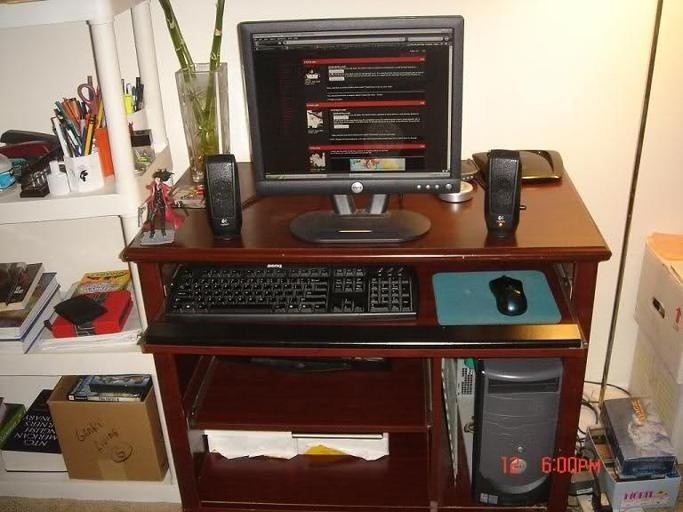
163;266;420;324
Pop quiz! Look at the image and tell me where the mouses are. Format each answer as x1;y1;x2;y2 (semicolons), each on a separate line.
489;275;528;317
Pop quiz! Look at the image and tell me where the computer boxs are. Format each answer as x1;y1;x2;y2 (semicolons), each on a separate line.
471;358;564;507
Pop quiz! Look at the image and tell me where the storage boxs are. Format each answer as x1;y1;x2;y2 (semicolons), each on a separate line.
627;328;683;462
46;375;167;482
586;425;682;512
633;232;682;382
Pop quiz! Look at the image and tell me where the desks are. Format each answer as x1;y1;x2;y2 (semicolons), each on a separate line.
121;153;609;511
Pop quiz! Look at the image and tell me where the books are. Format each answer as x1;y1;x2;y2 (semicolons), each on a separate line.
53;290;132;336
0;389;67;472
70;271;130;296
0;399;27;444
66;376;150;401
0;262;63;355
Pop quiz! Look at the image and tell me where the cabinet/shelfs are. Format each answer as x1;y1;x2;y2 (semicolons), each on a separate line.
1;0;181;508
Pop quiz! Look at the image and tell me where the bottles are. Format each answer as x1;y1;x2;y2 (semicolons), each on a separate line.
47;160;71;197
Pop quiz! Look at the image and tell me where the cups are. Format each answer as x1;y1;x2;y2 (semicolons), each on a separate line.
91;127;113;177
67;154;103;193
128;109;148;131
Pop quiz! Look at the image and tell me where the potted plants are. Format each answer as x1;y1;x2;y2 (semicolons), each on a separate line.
160;2;230;183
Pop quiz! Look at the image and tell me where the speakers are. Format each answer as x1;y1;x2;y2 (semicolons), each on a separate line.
483;149;522;238
202;152;243;241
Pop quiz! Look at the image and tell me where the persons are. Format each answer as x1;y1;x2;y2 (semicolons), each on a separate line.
141;169;184;245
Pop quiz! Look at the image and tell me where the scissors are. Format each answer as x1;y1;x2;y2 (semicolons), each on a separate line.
78;84;101;125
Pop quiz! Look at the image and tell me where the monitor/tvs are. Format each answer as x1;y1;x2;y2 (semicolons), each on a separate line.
236;14;466;243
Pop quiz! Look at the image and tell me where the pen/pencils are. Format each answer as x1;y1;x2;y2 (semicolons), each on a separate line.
52;98;94;158
121;77;144;114
88;76;93;100
96;100;103;129
